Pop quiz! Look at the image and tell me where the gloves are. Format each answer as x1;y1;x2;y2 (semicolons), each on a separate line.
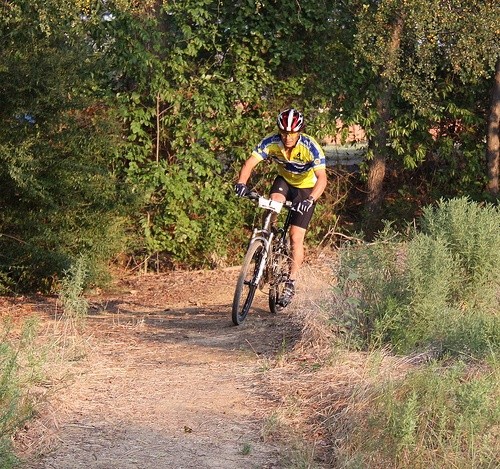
233;183;249;198
298;195;316;213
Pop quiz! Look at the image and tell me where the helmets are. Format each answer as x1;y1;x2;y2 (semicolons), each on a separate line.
277;108;303;133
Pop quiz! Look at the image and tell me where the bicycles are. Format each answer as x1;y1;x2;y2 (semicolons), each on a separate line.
231;190;310;326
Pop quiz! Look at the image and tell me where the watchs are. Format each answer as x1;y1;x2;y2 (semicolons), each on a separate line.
306;195;315;203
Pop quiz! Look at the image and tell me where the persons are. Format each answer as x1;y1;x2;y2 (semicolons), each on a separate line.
234;109;327;308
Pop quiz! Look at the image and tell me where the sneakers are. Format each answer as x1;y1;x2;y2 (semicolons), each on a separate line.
281;283;294;306
